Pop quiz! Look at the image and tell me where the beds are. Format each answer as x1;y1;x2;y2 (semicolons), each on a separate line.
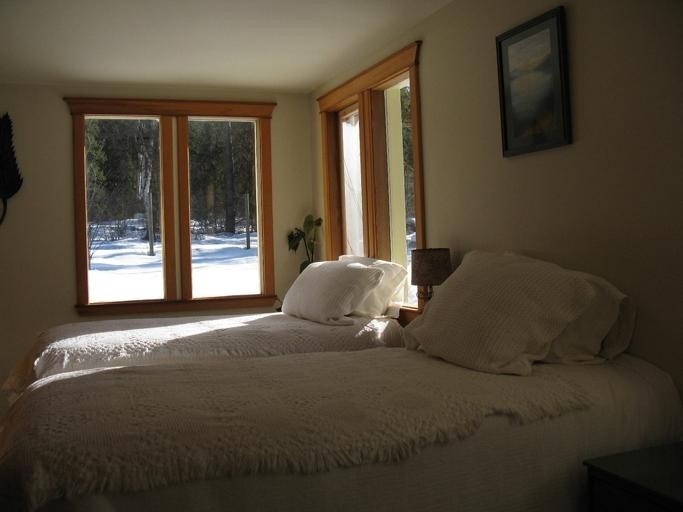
11;344;679;510
27;307;407;380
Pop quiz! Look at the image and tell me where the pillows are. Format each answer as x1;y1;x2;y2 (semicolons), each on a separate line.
399;240;636;379
284;251;407;325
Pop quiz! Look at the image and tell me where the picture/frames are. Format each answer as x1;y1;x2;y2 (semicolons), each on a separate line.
494;7;574;157
